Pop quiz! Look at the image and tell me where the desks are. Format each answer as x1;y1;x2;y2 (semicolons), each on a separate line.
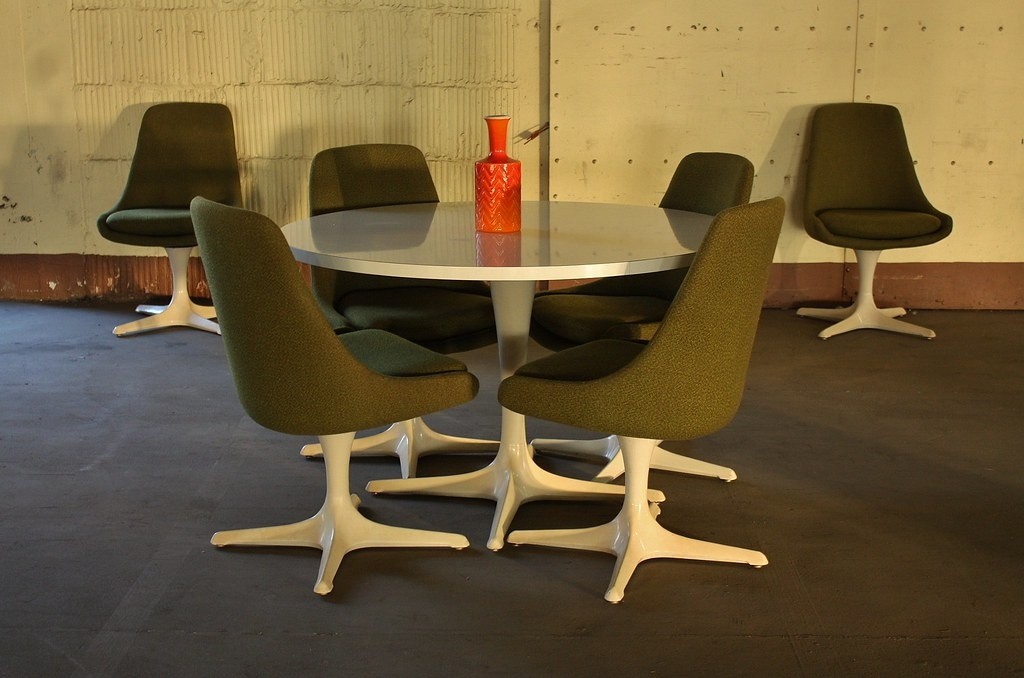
276;200;722;554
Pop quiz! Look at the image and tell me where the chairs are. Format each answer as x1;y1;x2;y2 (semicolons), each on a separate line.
190;195;480;595
509;196;787;603
299;142;540;483
97;101;244;337
797;103;952;340
525;152;756;484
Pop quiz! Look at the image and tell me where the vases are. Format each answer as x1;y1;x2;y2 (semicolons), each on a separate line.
473;115;522;234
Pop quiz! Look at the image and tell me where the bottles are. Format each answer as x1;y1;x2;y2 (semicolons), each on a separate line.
475;115;522;233
